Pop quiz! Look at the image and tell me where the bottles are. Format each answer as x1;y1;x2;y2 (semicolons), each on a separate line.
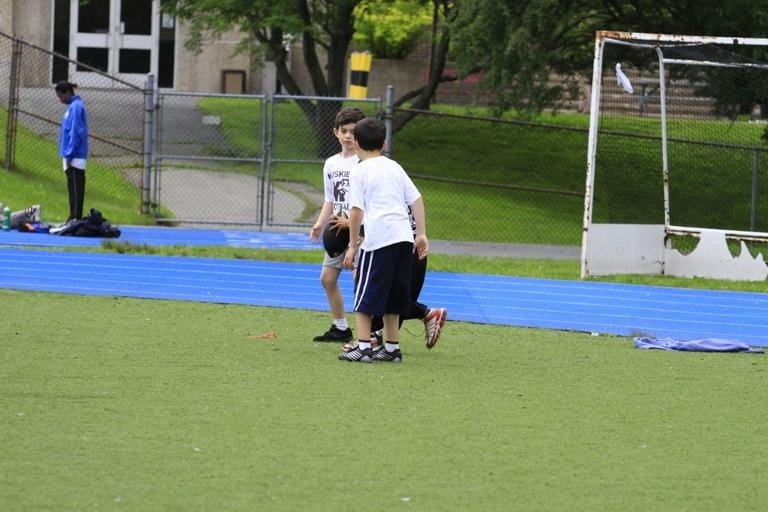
0;202;11;232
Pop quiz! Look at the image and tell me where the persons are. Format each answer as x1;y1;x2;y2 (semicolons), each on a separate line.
328;160;447;348
338;116;430;364
308;106;388;345
56;83;88;222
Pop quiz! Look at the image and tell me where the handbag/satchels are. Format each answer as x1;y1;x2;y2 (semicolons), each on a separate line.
71;209;121;238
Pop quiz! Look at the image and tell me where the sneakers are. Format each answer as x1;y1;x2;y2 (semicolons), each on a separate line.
313;325;352;342
339;331;402;362
422;308;447;348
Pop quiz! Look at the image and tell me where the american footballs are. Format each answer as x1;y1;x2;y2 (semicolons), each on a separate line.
323;211;350;258
